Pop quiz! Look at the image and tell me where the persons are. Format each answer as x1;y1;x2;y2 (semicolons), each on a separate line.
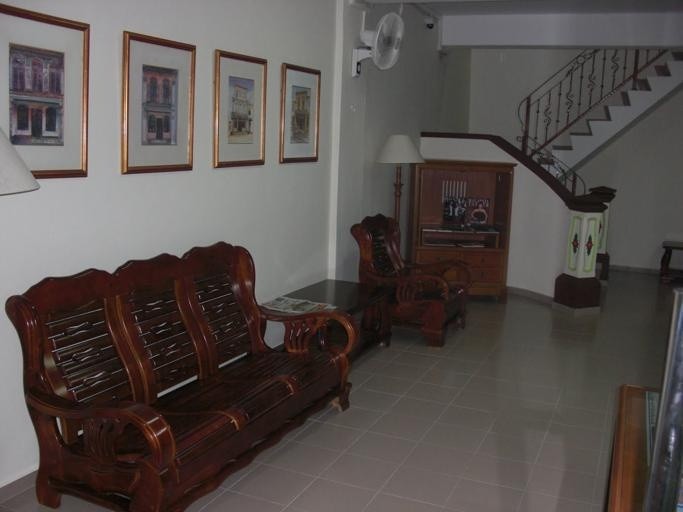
446;200;453;221
454;203;461;221
471;201;488;223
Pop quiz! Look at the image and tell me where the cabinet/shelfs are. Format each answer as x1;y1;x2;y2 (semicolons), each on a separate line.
604;288;683;512
411;160;518;304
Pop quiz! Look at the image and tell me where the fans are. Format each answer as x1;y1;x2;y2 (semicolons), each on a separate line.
351;13;404;79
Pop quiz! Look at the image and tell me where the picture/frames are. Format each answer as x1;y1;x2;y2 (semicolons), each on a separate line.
280;62;322;164
123;30;196;173
0;3;90;178
214;49;266;169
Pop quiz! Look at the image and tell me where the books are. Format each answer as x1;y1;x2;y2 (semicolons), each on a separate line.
423;241;490;248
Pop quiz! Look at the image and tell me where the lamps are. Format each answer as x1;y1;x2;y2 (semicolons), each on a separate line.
376;135;425;227
0;128;41;196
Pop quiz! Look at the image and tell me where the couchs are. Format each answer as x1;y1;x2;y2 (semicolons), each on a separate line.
351;213;480;347
5;241;359;512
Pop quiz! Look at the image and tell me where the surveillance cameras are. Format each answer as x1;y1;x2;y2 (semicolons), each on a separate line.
424;17;436;29
